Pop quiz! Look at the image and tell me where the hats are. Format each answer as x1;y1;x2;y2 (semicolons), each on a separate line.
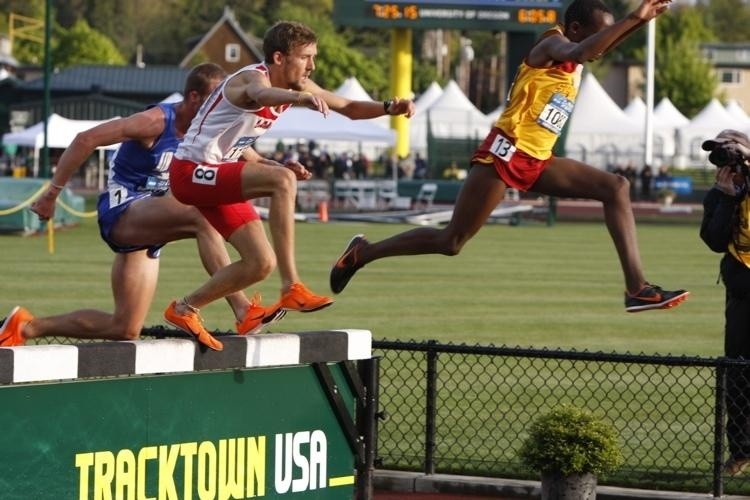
701;129;750;151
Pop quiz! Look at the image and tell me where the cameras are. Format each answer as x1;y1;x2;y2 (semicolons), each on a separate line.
709;144;749;173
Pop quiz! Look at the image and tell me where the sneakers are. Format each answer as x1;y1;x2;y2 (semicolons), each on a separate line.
0;303;33;346
163;299;225;353
623;281;689;314
329;233;374;295
277;281;333;313
236;300;288;337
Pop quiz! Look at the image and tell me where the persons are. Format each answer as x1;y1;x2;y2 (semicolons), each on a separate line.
659;166;670;176
3;146;34;177
0;60;316;347
697;128;750;483
328;0;691;314
159;13;417;355
266;138;369;183
375;151;427;180
640;163;653;201
623;160;639;201
613;163;624;176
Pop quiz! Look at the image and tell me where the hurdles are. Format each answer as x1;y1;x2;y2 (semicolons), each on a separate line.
0;328;380;500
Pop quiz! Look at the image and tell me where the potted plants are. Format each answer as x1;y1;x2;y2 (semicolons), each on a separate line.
516;403;620;500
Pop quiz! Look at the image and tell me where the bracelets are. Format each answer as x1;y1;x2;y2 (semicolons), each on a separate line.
384;100;393;115
